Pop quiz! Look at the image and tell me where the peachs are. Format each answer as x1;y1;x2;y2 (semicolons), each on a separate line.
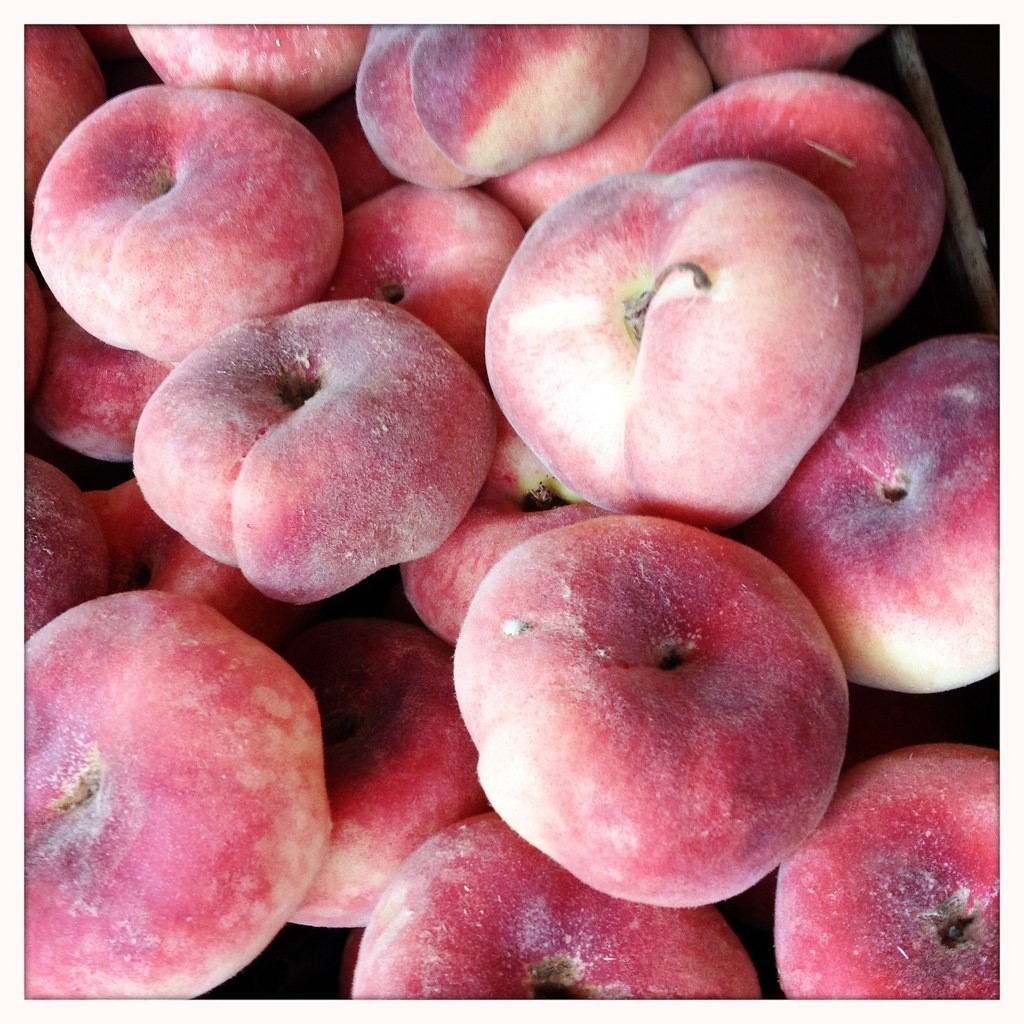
24;24;1000;1000
